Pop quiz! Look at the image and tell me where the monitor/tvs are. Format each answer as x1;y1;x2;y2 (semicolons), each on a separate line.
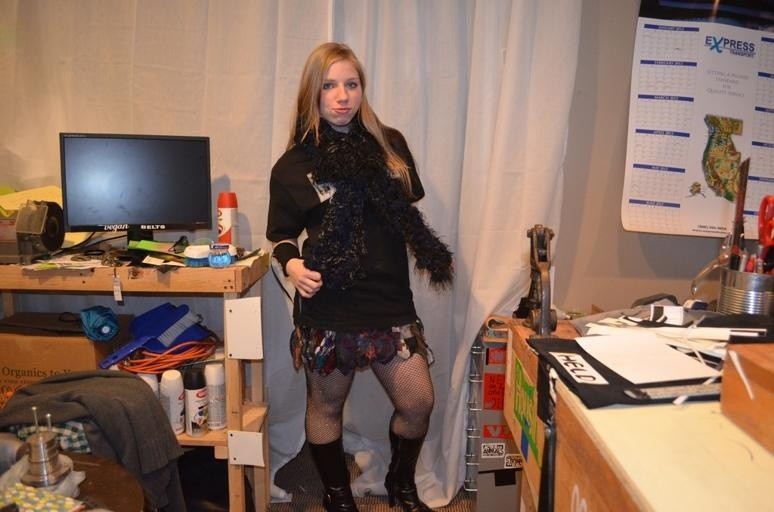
57;131;213;261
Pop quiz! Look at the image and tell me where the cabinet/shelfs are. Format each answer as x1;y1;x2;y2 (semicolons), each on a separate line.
502;312;774;512
466;321;526;512
0;250;274;512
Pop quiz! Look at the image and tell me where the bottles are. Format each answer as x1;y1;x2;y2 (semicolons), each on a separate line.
158;370;185;434
215;192;237;249
203;364;225;431
183;367;207;438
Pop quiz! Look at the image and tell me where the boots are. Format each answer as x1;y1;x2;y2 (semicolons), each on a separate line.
306;431;360;512
382;426;438;512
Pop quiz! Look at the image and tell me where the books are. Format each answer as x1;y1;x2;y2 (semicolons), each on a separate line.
693;313;770;341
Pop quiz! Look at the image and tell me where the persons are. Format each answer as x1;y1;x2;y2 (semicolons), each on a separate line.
266;42;456;512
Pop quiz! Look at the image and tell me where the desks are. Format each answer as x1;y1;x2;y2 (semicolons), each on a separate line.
0;446;147;512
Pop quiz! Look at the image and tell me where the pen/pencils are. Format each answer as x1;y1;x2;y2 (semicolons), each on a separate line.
729;246;765;274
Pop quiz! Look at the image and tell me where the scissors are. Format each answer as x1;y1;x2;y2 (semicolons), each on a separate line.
757;196;774;253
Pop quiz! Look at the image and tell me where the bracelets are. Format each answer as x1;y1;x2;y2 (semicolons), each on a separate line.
272;243;300;278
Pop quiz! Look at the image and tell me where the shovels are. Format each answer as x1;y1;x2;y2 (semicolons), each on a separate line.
99;303;212;370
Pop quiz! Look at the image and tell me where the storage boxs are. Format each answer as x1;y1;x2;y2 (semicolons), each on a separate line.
0;309;137;395
719;339;774;456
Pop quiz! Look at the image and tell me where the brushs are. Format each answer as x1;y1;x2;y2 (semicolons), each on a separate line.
107;304;201;363
